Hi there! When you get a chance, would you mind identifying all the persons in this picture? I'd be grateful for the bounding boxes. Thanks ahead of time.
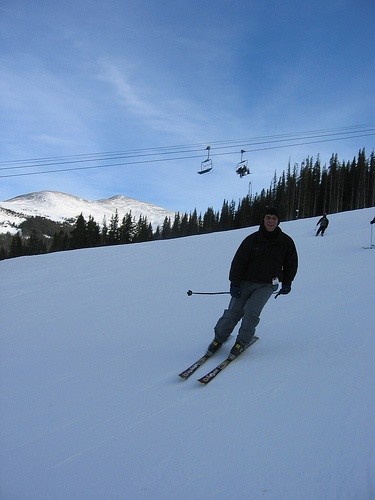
[237,165,252,176]
[206,206,298,358]
[316,214,329,236]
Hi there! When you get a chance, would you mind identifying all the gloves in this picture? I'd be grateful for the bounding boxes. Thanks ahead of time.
[279,281,291,295]
[230,284,241,298]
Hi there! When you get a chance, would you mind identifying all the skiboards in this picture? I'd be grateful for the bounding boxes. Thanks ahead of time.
[360,240,375,250]
[174,332,259,387]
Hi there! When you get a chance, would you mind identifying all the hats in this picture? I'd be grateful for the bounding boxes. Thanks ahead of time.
[262,206,280,225]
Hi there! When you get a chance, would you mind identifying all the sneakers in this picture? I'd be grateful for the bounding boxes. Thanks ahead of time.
[230,341,245,357]
[208,338,222,353]
